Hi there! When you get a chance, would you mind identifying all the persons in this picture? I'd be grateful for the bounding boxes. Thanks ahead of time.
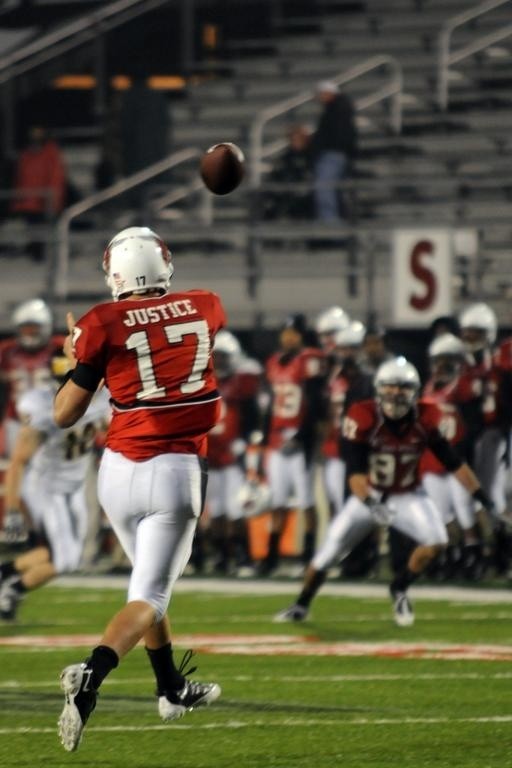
[294,74,357,225]
[10,117,64,262]
[1,223,511,752]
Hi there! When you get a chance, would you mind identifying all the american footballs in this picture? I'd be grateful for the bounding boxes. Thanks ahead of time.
[200,143,245,195]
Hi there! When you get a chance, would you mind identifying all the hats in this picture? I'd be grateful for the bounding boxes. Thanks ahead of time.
[279,313,306,334]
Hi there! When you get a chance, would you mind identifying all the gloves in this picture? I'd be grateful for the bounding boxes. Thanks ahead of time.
[369,491,397,525]
[0,513,29,545]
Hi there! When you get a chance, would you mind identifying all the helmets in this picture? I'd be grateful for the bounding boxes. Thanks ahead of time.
[212,330,239,379]
[238,479,273,518]
[11,298,52,353]
[374,356,423,416]
[428,302,498,380]
[102,225,175,302]
[314,305,386,358]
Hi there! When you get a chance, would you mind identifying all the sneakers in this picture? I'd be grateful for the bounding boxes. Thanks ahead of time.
[0,560,23,620]
[389,583,414,627]
[57,661,99,751]
[158,681,221,724]
[183,554,376,580]
[272,603,309,624]
[429,543,511,584]
